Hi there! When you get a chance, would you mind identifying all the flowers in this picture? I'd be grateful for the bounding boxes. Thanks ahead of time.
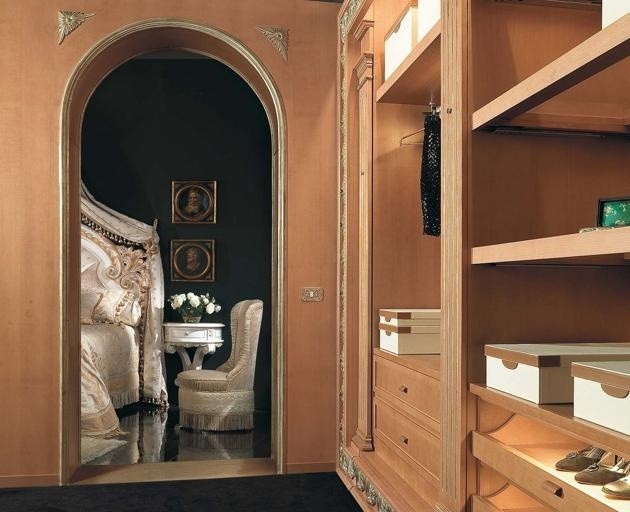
[167,292,222,317]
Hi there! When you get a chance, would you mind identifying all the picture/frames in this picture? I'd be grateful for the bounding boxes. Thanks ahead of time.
[171,179,217,225]
[170,240,215,282]
[598,197,630,225]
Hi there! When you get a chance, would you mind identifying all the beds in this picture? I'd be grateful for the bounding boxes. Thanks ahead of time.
[78,177,169,437]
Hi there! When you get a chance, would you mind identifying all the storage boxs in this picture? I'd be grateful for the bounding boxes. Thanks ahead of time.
[380,308,440,327]
[485,342,630,404]
[379,322,440,355]
[570,361,630,438]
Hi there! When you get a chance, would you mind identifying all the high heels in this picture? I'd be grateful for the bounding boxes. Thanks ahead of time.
[554,446,608,471]
[575,450,630,484]
[602,473,630,499]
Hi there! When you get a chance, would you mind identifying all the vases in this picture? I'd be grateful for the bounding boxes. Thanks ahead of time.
[182,318,201,322]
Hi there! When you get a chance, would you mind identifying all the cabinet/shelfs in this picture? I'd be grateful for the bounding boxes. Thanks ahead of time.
[452,0,630,512]
[336,0,450,512]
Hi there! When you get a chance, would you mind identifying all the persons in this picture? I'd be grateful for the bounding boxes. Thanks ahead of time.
[184,248,203,275]
[183,190,204,216]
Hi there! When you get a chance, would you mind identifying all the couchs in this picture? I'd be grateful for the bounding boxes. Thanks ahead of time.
[174,298,264,431]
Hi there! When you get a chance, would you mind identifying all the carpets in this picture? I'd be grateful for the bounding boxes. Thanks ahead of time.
[80,437,129,463]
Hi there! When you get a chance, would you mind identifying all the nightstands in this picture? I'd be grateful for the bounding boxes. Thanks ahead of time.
[163,319,225,386]
